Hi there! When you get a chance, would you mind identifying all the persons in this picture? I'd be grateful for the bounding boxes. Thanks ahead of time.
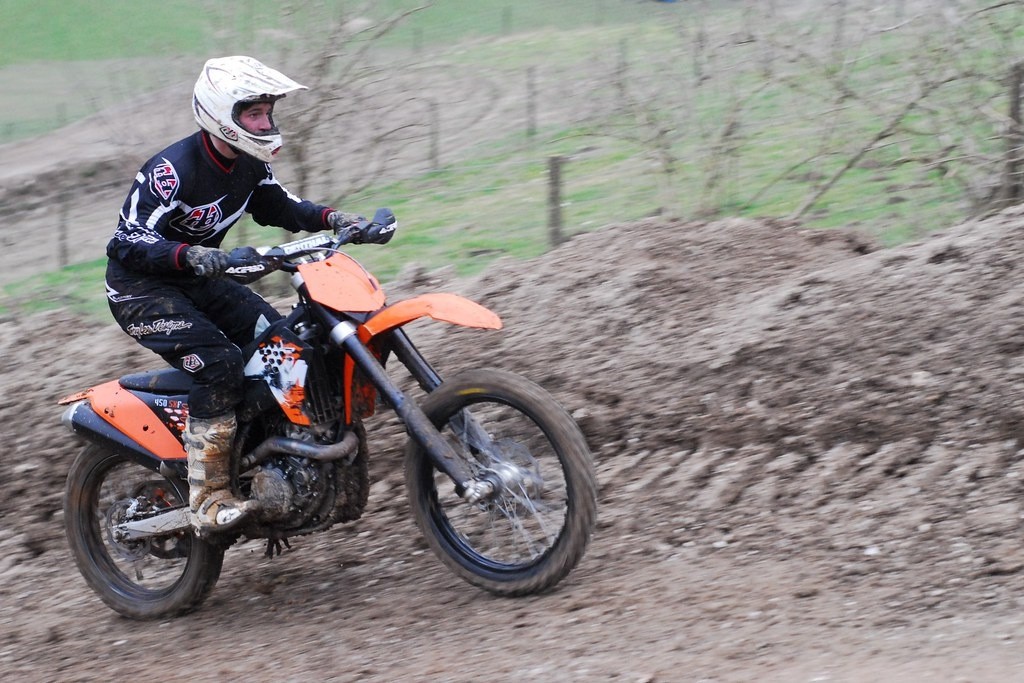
[105,56,368,530]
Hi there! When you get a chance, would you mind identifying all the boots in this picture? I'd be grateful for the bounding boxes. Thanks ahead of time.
[185,408,261,533]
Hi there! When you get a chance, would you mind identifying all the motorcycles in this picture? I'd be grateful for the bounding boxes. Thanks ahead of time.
[47,220,601,623]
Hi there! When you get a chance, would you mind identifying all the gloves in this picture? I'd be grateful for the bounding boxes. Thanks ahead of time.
[186,245,230,281]
[326,211,370,246]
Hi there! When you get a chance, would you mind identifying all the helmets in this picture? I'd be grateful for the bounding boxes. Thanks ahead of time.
[191,55,309,164]
